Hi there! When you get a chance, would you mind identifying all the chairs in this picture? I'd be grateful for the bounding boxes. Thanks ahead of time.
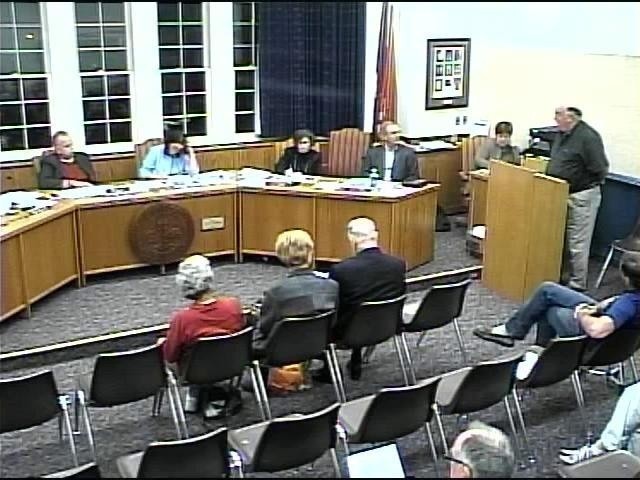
[593,212,640,288]
[459,135,496,202]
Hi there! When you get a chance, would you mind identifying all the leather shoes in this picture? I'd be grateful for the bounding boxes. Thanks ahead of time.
[346,359,362,380]
[557,444,609,466]
[472,323,515,347]
[310,367,344,384]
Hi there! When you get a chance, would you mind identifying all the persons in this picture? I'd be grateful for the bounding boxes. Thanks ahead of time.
[471,249,640,348]
[361,120,420,181]
[164,254,244,420]
[274,128,320,177]
[37,131,96,190]
[239,227,341,393]
[557,380,640,466]
[474,121,522,168]
[544,104,609,293]
[449,422,514,479]
[137,128,199,180]
[311,216,406,383]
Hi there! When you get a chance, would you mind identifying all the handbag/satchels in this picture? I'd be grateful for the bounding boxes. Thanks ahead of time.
[200,385,241,420]
[267,363,306,396]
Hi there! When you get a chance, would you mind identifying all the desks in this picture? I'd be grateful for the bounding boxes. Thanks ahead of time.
[466,167,490,256]
[417,149,469,215]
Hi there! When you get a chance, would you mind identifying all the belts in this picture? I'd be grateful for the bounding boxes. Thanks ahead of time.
[568,180,600,195]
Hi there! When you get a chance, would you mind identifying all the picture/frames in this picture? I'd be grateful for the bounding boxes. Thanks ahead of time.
[425,37,472,110]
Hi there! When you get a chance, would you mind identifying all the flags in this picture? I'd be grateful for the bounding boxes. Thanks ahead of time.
[372,2,398,140]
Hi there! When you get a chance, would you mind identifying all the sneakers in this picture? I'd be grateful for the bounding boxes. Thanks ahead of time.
[184,389,198,412]
